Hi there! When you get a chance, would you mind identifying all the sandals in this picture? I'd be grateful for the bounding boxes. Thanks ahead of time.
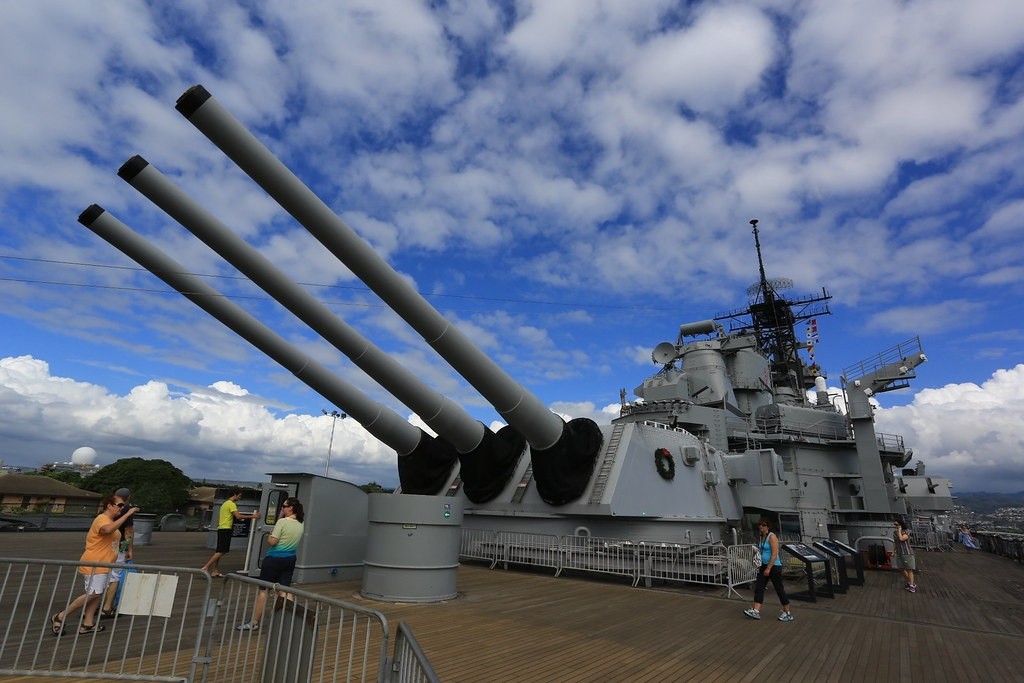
[101,609,121,618]
[236,621,259,630]
[51,611,66,636]
[79,624,105,634]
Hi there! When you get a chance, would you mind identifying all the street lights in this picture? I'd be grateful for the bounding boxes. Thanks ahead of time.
[322,409,348,479]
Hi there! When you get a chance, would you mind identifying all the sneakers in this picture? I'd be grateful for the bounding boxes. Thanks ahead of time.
[778,612,794,621]
[743,608,760,619]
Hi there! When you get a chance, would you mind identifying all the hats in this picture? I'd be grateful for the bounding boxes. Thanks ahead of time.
[115,488,130,499]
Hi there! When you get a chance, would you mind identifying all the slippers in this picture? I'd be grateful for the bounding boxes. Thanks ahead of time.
[211,573,225,578]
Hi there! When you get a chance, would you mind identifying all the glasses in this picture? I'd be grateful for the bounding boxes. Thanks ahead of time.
[894,523,900,526]
[283,505,294,509]
[110,503,124,507]
[759,523,767,527]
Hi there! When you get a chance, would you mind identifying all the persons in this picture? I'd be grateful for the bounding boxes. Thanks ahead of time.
[50,495,140,636]
[743,517,794,621]
[237,497,305,630]
[891,519,917,592]
[80,488,134,619]
[201,488,262,578]
[960,525,977,551]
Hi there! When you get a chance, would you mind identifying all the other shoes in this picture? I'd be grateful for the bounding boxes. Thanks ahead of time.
[905,582,916,593]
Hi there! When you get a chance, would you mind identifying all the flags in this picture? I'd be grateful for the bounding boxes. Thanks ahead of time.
[806,319,819,376]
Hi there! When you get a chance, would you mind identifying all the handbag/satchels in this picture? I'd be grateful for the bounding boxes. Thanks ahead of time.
[113,560,138,609]
